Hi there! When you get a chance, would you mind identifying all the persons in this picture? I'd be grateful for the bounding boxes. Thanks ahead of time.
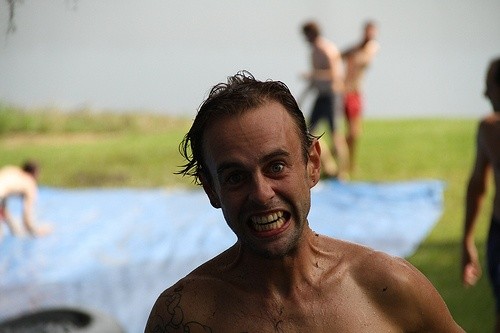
[462,57,500,333]
[339,18,379,182]
[144,70,468,333]
[1,158,53,238]
[298,21,350,183]
